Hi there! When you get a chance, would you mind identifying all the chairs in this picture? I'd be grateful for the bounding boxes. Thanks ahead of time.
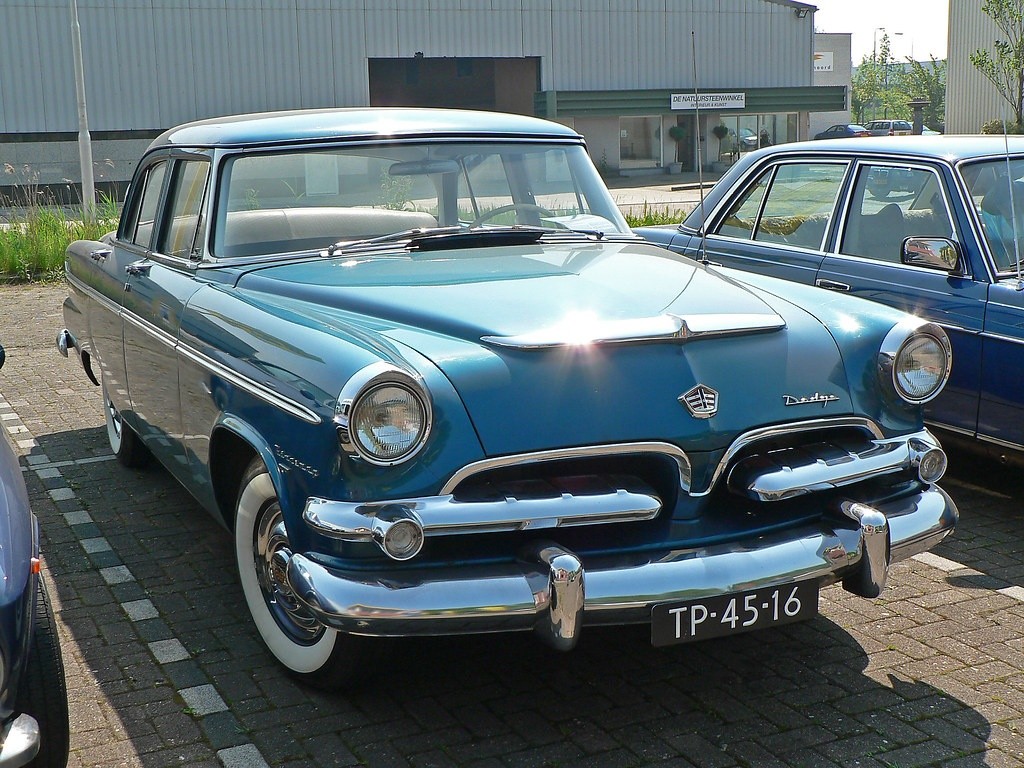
[864,203,908,262]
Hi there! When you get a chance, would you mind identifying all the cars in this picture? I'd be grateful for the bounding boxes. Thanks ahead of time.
[813,120,942,140]
[54,104,962,697]
[727,128,763,152]
[627,131,1024,501]
[1,341,72,768]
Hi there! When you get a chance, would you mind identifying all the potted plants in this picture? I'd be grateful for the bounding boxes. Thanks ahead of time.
[667,124,687,174]
[713,125,729,173]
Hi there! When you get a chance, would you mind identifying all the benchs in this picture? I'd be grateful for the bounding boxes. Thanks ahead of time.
[136,207,438,258]
[761,193,950,263]
